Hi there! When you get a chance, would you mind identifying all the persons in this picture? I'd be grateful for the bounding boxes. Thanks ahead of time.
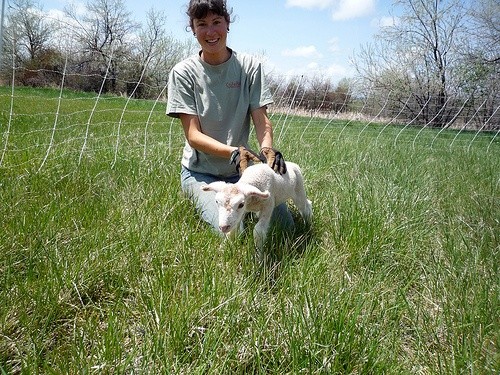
[166,0,294,246]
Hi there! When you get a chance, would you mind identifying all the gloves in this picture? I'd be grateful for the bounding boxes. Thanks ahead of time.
[228,145,261,179]
[258,147,287,175]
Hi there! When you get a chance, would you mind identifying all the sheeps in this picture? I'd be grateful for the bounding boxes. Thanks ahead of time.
[201,160,312,251]
